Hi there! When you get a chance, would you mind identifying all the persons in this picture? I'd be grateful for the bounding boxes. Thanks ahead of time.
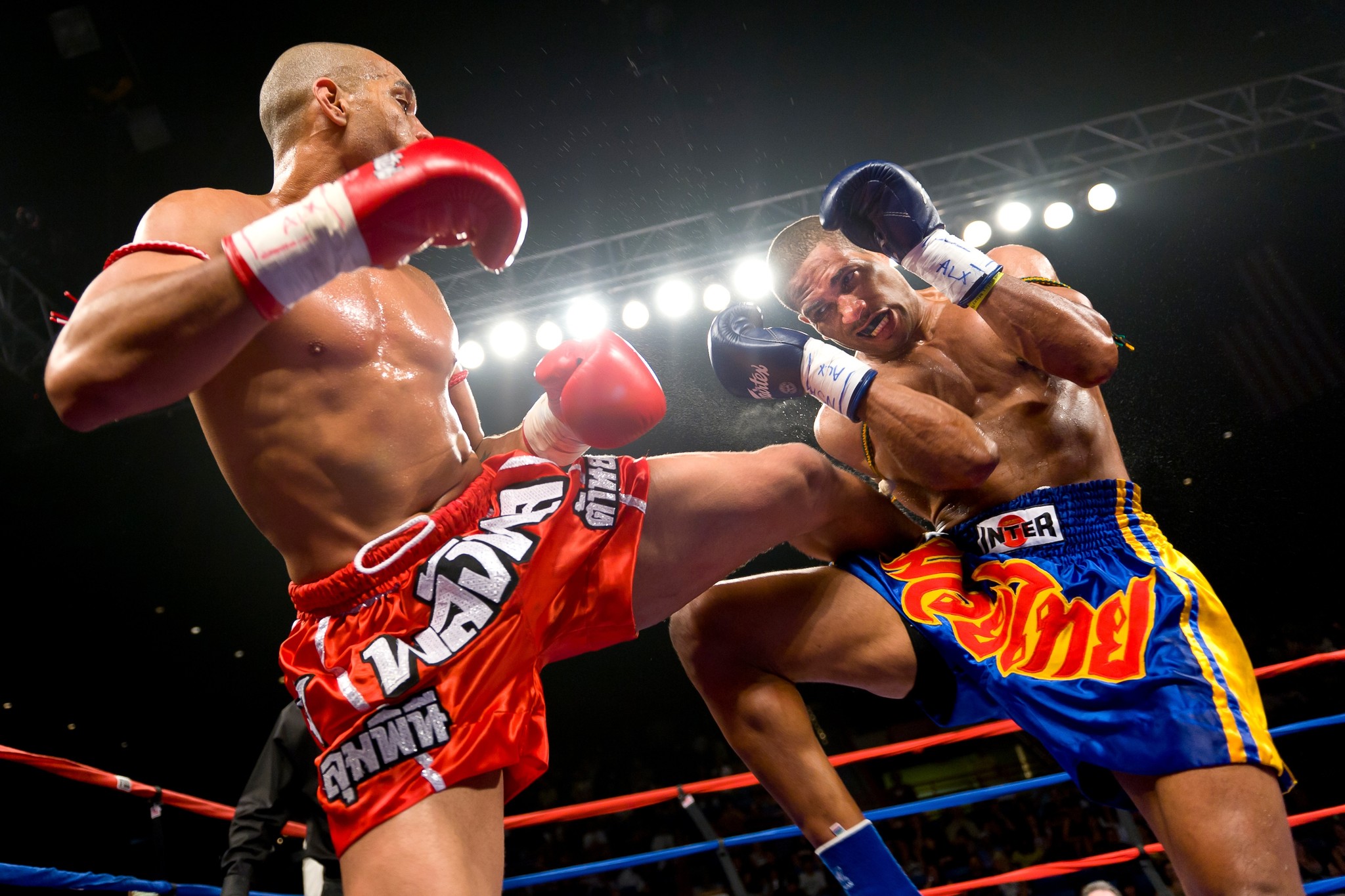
[505,608,1345,896]
[1082,880,1121,896]
[668,158,1307,896]
[43,41,929,896]
[221,699,343,896]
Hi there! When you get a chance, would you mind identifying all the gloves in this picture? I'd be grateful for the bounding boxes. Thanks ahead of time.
[519,329,668,468]
[709,304,879,424]
[819,160,1003,306]
[223,137,526,319]
